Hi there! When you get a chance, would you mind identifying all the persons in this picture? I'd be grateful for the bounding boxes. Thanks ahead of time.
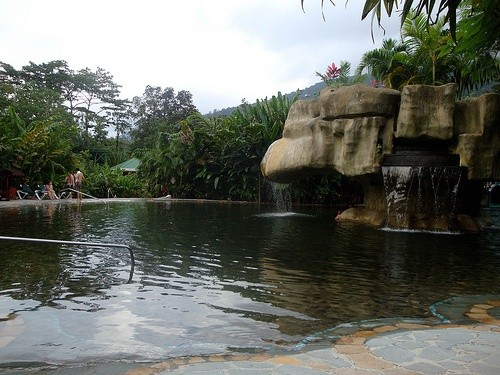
[45,181,55,200]
[64,171,74,199]
[75,168,84,200]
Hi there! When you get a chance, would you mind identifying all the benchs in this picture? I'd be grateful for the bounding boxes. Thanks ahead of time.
[17,184,66,200]
